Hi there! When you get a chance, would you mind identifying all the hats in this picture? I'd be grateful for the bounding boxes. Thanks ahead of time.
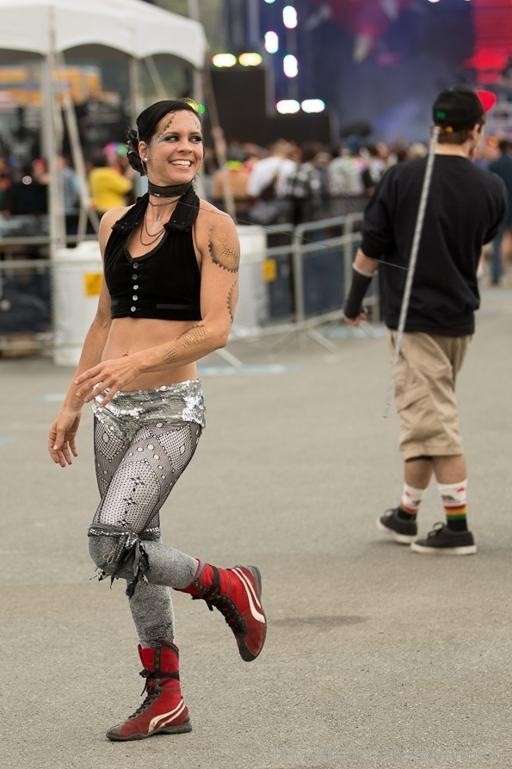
[432,86,497,132]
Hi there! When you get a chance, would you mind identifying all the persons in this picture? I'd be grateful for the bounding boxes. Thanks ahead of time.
[341,86,512,559]
[0,135,510,289]
[46,101,269,741]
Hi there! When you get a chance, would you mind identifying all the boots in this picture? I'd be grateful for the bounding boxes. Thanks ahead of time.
[107,637,192,740]
[172,559,267,661]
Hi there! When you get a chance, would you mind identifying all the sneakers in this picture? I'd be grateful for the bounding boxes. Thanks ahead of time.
[411,522,477,555]
[376,508,417,544]
